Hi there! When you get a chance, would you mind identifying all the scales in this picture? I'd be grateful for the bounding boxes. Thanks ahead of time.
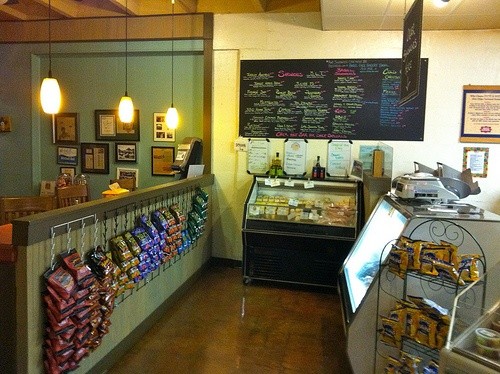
[394,171,461,204]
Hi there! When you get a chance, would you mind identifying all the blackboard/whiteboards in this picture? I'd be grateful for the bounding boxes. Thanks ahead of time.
[238,58,429,142]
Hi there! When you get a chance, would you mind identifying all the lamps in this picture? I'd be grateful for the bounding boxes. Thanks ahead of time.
[164,1,181,131]
[38,1,63,115]
[117,1,135,123]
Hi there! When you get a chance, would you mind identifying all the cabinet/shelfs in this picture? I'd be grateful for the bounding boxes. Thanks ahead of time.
[371,218,487,373]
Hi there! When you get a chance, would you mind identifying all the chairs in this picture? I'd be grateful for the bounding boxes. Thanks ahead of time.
[110,177,135,193]
[55,183,91,209]
[1,196,59,224]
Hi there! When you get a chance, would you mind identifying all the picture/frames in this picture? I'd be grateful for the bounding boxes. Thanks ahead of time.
[60,167,76,177]
[94,109,141,142]
[151,146,175,176]
[115,142,137,162]
[40,180,56,196]
[51,113,79,145]
[56,147,79,166]
[116,168,139,188]
[152,111,176,143]
[80,142,110,174]
[0,114,12,132]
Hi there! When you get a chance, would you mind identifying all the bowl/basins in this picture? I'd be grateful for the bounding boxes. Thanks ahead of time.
[474,341,500,360]
[475,327,500,347]
